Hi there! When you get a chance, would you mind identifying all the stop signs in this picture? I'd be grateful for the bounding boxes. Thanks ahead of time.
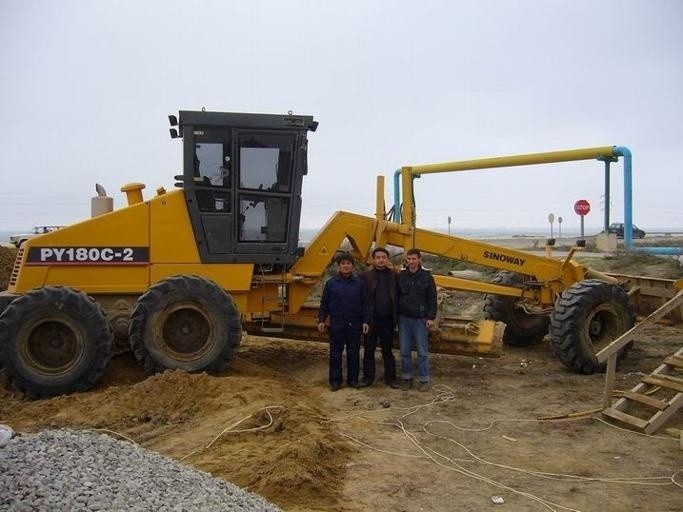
[574,199,590,216]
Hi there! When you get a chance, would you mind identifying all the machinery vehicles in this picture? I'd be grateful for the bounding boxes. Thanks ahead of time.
[0,108,682,401]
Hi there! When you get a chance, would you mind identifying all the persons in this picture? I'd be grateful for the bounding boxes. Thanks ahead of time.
[397,248,437,392]
[317,254,369,391]
[357,248,399,389]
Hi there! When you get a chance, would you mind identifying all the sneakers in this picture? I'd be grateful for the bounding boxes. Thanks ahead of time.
[359,379,370,386]
[331,383,339,391]
[386,380,399,388]
[420,382,429,391]
[402,380,412,391]
[350,380,360,388]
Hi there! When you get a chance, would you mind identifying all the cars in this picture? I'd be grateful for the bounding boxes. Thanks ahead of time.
[8,224,67,248]
[607,224,645,239]
